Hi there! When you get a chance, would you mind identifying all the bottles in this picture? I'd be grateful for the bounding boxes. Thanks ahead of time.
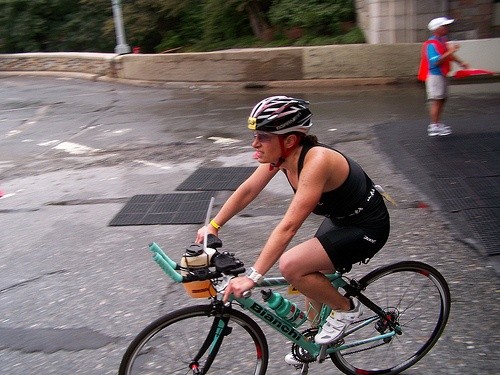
[180,242,216,299]
[260,288,307,327]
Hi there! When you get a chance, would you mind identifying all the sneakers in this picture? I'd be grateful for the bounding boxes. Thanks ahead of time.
[314,296,364,344]
[284,348,308,365]
[428,123,452,136]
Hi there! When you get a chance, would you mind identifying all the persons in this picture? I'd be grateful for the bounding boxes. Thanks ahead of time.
[417,17,468,135]
[195,96,390,365]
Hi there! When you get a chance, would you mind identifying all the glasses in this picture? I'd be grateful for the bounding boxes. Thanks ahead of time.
[252,132,274,142]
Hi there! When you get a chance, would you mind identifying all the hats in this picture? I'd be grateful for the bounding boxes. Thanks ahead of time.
[427,17,454,31]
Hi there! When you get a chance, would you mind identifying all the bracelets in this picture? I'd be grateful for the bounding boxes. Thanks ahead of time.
[210,220,221,229]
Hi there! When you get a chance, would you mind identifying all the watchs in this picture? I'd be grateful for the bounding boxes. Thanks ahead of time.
[245,267,264,285]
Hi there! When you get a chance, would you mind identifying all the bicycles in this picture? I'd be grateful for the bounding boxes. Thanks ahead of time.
[118,233,451,375]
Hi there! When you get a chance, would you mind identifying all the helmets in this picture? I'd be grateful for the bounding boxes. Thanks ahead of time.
[248,95,312,134]
